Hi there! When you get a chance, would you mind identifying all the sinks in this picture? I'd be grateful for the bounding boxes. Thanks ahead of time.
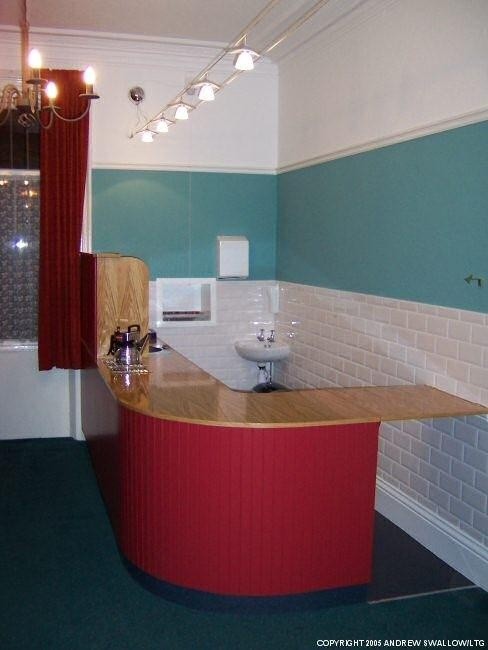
[235,339,289,361]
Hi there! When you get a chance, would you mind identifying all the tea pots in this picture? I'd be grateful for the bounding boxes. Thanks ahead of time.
[113,342,141,366]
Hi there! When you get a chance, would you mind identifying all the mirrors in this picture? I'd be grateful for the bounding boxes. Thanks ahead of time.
[154,277,217,327]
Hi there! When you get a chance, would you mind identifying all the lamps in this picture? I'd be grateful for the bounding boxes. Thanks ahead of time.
[135,34,261,142]
[1,0,101,132]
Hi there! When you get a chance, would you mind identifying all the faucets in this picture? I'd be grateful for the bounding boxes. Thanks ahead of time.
[267,330,276,342]
[256,329,264,341]
